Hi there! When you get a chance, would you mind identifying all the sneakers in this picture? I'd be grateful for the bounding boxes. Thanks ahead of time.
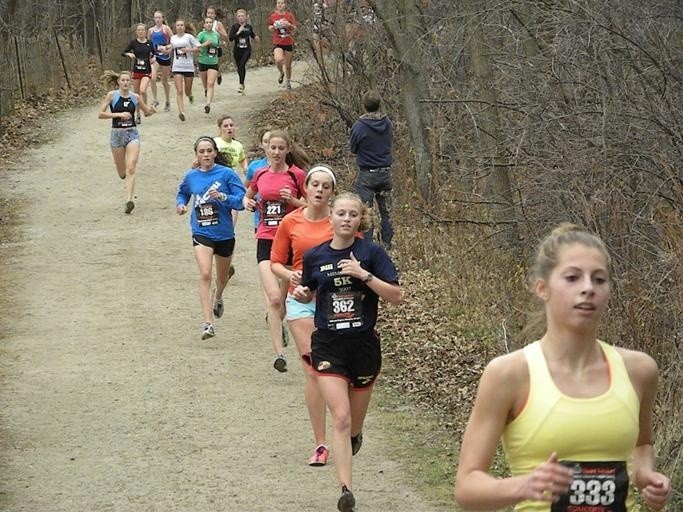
[309,444,329,467]
[286,82,291,90]
[216,74,223,85]
[350,425,364,456]
[278,72,284,84]
[237,84,246,93]
[204,89,208,97]
[135,111,143,124]
[189,96,195,104]
[228,264,236,280]
[211,287,225,319]
[150,100,160,112]
[178,111,186,121]
[265,312,290,348]
[205,104,211,114]
[337,483,356,512]
[201,321,215,341]
[125,199,135,215]
[272,353,288,374]
[163,101,171,112]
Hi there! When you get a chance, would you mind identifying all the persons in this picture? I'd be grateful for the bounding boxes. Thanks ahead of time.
[455,220,671,512]
[270,163,367,466]
[229,8,260,93]
[304,0,331,71]
[120,5,230,120]
[176,129,247,341]
[292,193,403,512]
[192,116,248,282]
[97,70,156,214]
[267,0,297,89]
